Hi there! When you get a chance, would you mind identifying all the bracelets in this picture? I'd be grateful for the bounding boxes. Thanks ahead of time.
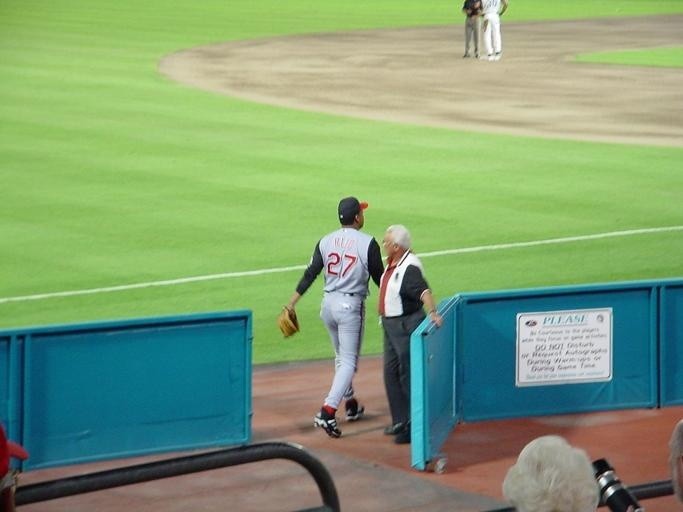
[428,309,437,315]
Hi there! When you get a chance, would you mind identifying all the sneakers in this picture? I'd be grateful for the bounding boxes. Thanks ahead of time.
[462,53,501,62]
[313,406,342,438]
[346,404,364,421]
[385,423,402,435]
[395,428,411,443]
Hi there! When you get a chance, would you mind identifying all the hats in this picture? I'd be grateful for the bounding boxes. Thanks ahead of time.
[338,197,369,220]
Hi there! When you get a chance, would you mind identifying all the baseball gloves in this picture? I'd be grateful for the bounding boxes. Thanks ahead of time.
[278,306,298,337]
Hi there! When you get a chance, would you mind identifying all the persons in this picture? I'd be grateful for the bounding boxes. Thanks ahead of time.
[463,0,483,59]
[501,435,601,512]
[626,421,683,511]
[480,0,509,61]
[378,224,443,443]
[290,197,385,439]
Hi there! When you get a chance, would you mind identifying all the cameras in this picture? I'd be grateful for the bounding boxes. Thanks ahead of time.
[592,456,646,512]
